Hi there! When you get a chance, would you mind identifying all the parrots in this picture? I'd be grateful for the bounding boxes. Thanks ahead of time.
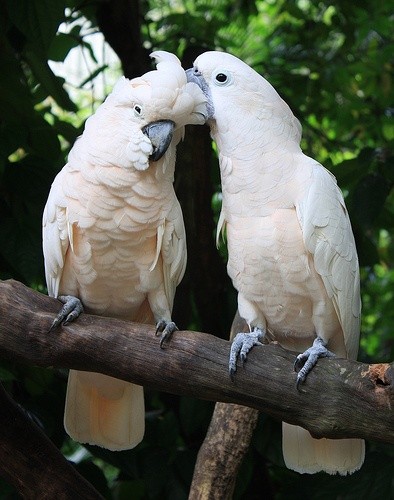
[42,51,208,452]
[185,49,365,475]
